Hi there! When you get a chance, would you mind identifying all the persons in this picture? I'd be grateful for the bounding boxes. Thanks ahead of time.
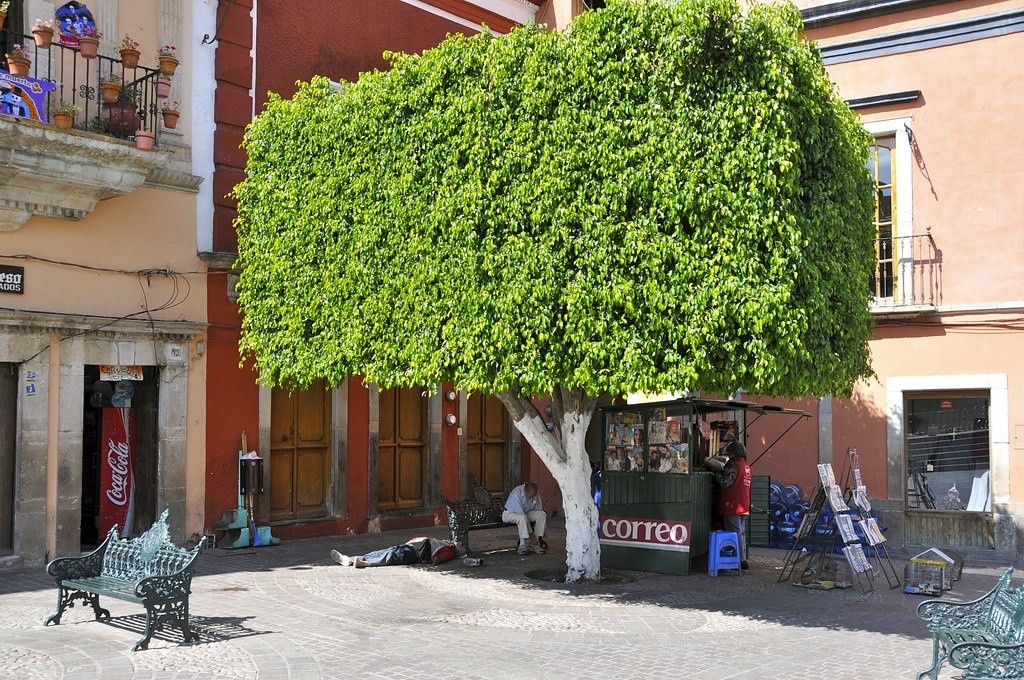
[650,450,689,473]
[606,449,644,471]
[634,429,644,445]
[666,421,681,444]
[648,424,659,444]
[941,488,964,510]
[716,442,752,570]
[331,537,456,567]
[609,424,630,445]
[502,482,548,554]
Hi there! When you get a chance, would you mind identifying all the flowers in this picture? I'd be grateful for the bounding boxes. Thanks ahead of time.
[95,70,144,107]
[81,25,103,41]
[156,46,177,59]
[118,34,140,50]
[35,18,55,27]
[100,72,121,85]
[161,98,182,111]
[2,44,32,66]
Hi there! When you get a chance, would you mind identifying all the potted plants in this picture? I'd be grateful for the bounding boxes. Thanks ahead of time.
[0,1,10,30]
[153,72,174,99]
[50,98,83,128]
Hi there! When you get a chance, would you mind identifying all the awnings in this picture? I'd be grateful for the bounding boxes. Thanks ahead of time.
[694,398,813,470]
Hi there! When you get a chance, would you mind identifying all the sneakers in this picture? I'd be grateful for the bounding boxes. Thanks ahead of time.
[528,543,546,555]
[519,544,527,555]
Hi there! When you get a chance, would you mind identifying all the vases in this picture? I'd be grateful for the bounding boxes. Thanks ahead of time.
[101,82,121,103]
[5,55,32,79]
[119,49,141,69]
[32,24,54,49]
[162,110,180,129]
[100,102,141,139]
[134,131,155,152]
[77,37,100,59]
[158,57,178,75]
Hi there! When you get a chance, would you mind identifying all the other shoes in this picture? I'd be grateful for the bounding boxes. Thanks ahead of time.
[740,561,748,570]
[354,557,362,569]
[330,550,352,566]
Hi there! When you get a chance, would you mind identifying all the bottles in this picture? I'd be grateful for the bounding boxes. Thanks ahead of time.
[768,482,884,556]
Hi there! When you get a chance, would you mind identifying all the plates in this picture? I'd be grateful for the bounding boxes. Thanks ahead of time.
[84,379,134,407]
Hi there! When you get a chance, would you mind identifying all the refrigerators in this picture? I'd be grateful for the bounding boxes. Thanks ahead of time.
[80,405,138,546]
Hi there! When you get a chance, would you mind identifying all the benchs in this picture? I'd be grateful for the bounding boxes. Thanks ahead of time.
[44,508,207,650]
[440,473,548,566]
[915,565,1024,680]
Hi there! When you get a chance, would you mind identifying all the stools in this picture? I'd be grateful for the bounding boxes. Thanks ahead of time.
[707,530,743,577]
